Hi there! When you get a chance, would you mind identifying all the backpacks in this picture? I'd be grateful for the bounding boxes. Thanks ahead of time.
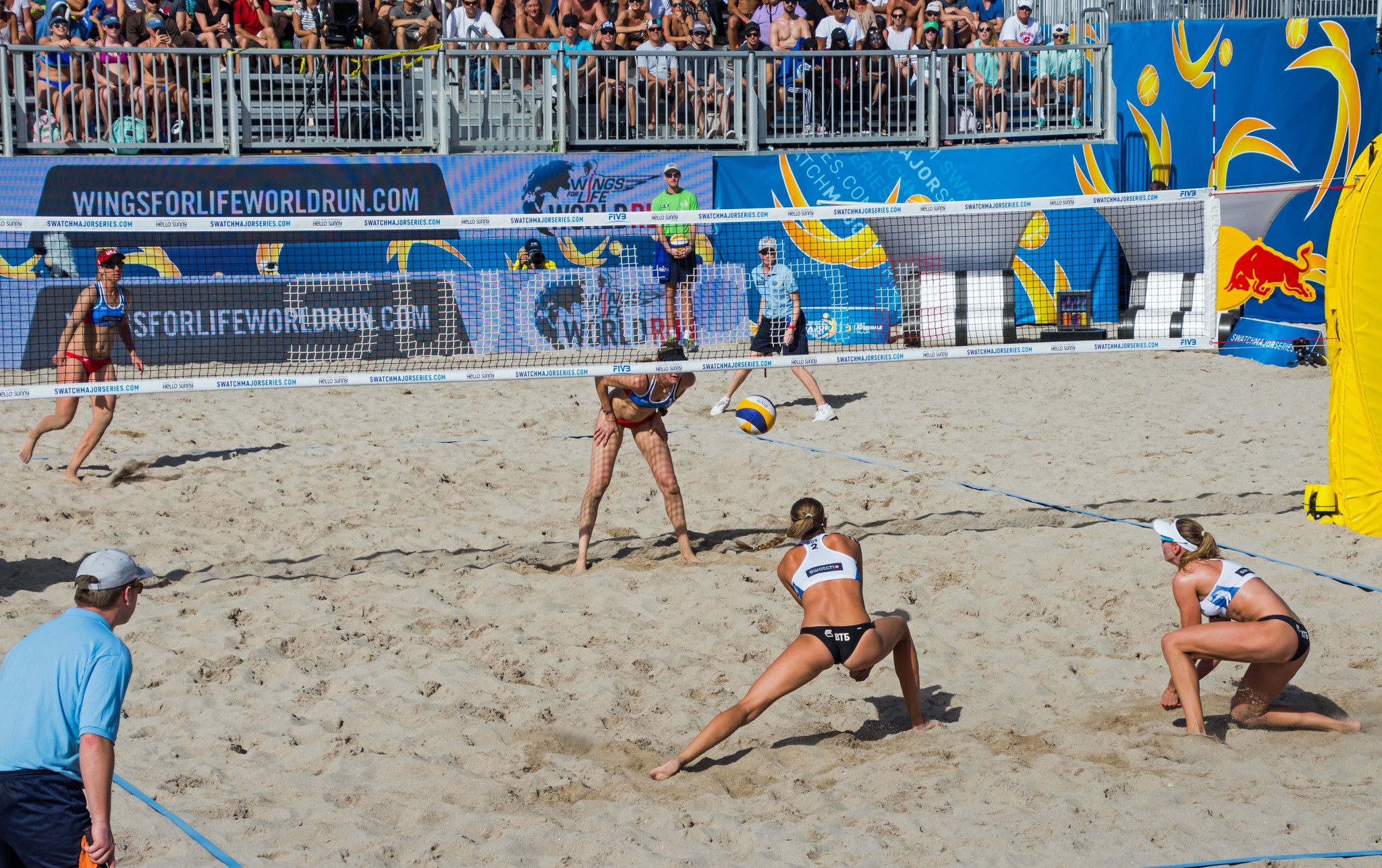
[953,105,979,133]
[108,117,145,155]
[26,110,68,155]
[467,58,500,90]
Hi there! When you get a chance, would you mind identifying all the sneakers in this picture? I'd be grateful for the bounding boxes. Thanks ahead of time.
[668,335,699,352]
[812,405,836,422]
[710,397,730,416]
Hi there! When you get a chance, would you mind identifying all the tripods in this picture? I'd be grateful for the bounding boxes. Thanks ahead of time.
[283,45,413,144]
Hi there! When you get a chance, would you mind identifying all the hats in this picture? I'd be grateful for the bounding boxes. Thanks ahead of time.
[102,16,120,25]
[758,236,775,251]
[524,238,542,252]
[562,14,579,25]
[1019,0,1033,9]
[1153,518,1197,552]
[600,21,616,30]
[833,0,849,8]
[745,21,760,32]
[146,19,165,29]
[924,21,939,31]
[692,21,708,34]
[97,250,126,271]
[1052,24,1068,34]
[75,548,154,590]
[648,19,662,28]
[832,28,848,39]
[664,162,680,173]
[50,15,68,26]
[925,2,941,12]
[658,348,688,361]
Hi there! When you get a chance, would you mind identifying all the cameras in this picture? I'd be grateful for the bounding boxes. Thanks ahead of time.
[523,249,547,266]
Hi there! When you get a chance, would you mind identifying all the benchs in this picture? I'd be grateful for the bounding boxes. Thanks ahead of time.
[0,0,1090,134]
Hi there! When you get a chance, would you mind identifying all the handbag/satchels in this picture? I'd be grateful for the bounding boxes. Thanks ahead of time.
[602,121,633,140]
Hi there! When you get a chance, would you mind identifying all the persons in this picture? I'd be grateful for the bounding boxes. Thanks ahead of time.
[1153,518,1366,737]
[647,498,946,784]
[0,547,154,868]
[1,0,1083,576]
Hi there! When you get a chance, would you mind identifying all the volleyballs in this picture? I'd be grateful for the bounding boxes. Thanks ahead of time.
[669,234,689,252]
[736,395,777,436]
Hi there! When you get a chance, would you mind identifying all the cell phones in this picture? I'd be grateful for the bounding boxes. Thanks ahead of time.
[159,27,166,38]
[412,30,422,38]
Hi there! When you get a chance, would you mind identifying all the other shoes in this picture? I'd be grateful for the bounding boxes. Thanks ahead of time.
[939,117,1081,146]
[404,68,437,79]
[275,63,290,82]
[599,121,736,139]
[767,99,893,135]
[502,79,510,90]
[220,66,235,74]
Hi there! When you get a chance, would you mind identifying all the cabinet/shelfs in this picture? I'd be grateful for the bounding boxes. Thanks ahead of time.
[1056,289,1094,331]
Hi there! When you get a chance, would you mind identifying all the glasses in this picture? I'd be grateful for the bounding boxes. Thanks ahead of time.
[565,23,578,28]
[666,173,680,178]
[746,32,759,38]
[760,250,775,255]
[629,0,641,3]
[870,36,882,41]
[92,6,102,11]
[102,260,124,269]
[54,7,67,12]
[869,29,881,34]
[1018,6,1031,12]
[464,0,477,6]
[149,0,160,3]
[122,582,144,594]
[53,22,68,28]
[892,14,904,18]
[1053,34,1066,38]
[107,24,120,29]
[785,0,796,5]
[672,3,684,8]
[835,5,847,10]
[924,29,936,34]
[603,30,615,34]
[927,12,940,16]
[649,27,663,32]
[979,26,991,32]
